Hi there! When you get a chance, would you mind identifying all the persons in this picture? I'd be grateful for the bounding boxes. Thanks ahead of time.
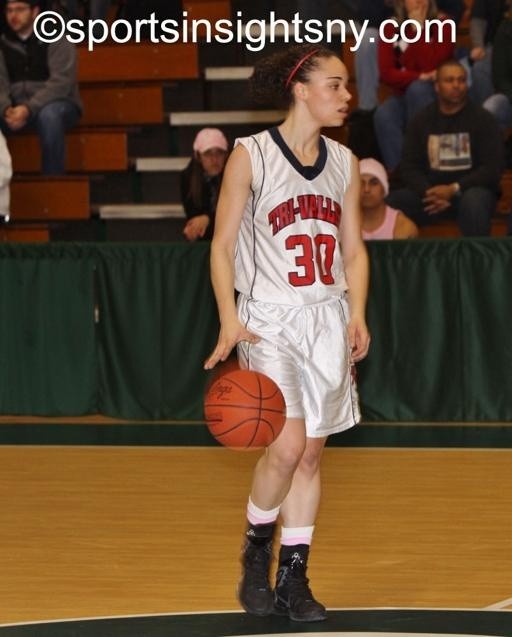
[0,0,87,176]
[349,156,422,239]
[382,57,507,236]
[199,39,375,626]
[48,0,189,42]
[348,1,511,188]
[177,126,232,242]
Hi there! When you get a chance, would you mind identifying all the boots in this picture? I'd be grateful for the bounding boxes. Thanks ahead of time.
[239,520,328,622]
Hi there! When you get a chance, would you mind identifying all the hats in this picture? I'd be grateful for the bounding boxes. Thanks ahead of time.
[358,157,390,199]
[192,127,228,155]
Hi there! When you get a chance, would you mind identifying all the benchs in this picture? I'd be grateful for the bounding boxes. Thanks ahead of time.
[0,2,512,242]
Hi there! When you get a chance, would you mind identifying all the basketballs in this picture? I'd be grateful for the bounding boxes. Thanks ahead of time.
[204,370,286,449]
